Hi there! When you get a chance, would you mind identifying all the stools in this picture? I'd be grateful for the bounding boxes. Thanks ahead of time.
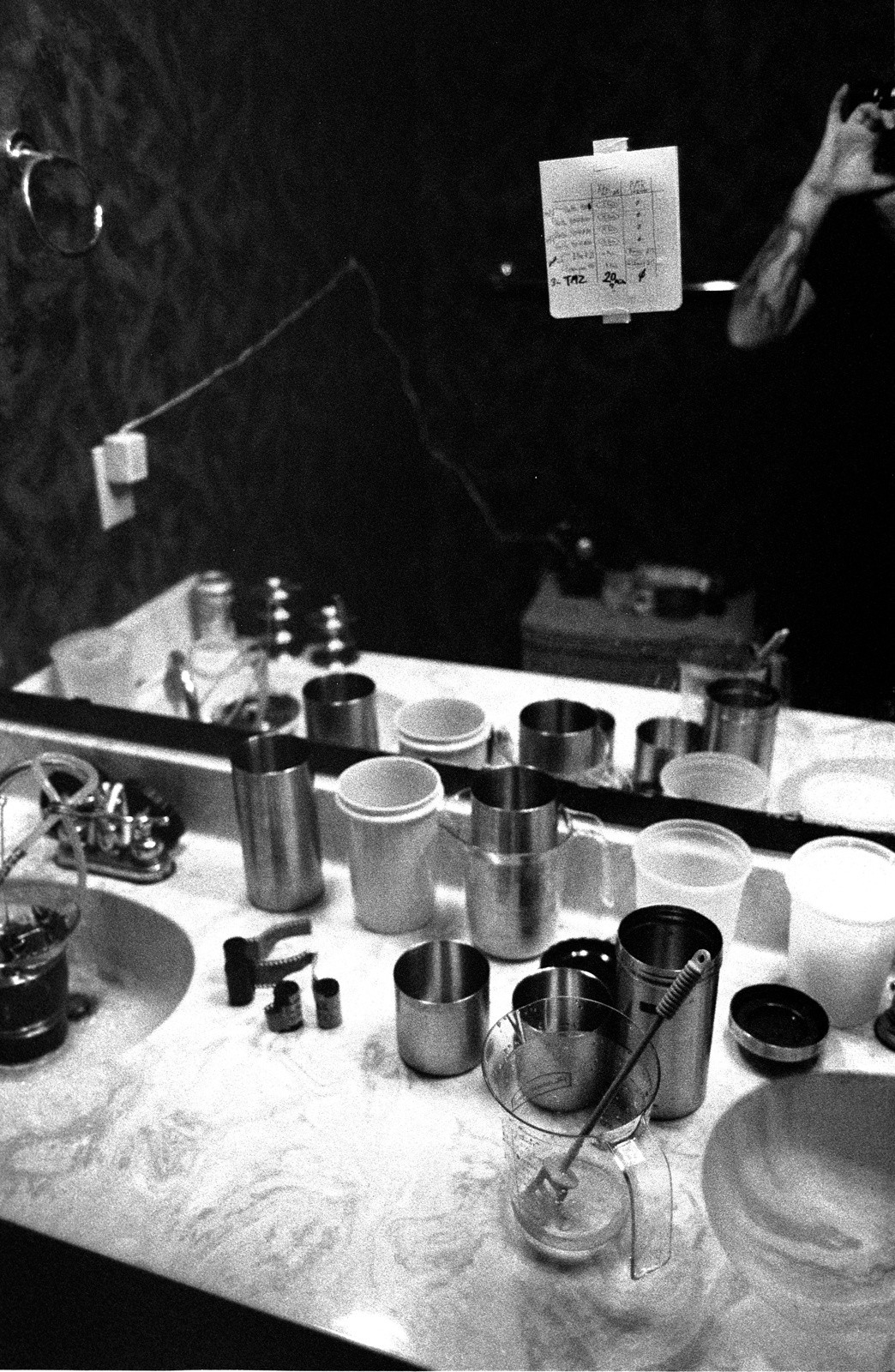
[519,559,767,693]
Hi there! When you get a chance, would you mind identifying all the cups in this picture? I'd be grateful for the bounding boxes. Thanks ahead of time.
[50,627,132,708]
[334,755,443,933]
[230,734,325,911]
[632,818,753,954]
[785,835,895,1030]
[519,698,616,772]
[613,904,724,1120]
[469,765,559,959]
[704,677,782,773]
[634,716,712,797]
[778,756,895,833]
[393,696,490,771]
[659,750,774,811]
[548,525,610,599]
[631,564,712,621]
[393,941,492,1077]
[511,967,612,1114]
[302,673,379,751]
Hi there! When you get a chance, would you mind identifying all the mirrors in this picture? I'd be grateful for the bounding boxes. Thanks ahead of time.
[0,0,895,861]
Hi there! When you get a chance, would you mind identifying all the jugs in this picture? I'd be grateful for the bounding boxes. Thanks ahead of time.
[438,787,614,959]
[678,636,782,728]
[481,996,673,1281]
[499,731,634,791]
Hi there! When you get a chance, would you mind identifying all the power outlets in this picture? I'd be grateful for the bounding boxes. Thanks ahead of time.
[88,442,139,534]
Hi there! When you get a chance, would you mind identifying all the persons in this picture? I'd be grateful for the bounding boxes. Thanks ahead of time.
[727,82,895,723]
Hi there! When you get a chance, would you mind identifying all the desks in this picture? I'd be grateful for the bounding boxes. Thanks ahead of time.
[0,793,895,1372]
[127,637,895,832]
